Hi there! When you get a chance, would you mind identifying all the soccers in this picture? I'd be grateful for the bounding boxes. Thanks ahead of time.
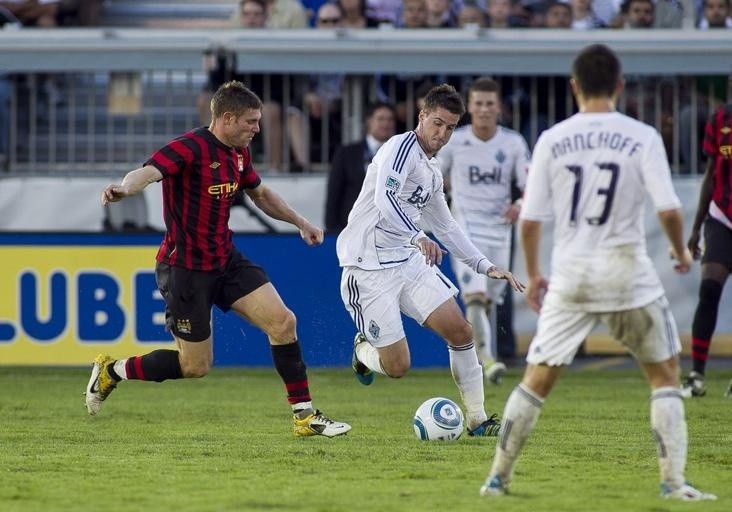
[414,397,464,440]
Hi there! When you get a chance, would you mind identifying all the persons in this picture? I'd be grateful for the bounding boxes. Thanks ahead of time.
[85,80,352,439]
[478,44,719,502]
[432,78,532,386]
[4,3,732,233]
[687,100,732,398]
[336,82,525,438]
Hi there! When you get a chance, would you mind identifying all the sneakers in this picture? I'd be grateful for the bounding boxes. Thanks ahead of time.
[483,360,507,388]
[478,471,513,498]
[675,369,707,400]
[658,480,719,504]
[466,413,503,438]
[352,329,377,387]
[292,409,353,438]
[82,352,118,415]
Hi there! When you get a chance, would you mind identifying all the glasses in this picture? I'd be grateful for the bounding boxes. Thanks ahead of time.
[318,18,343,25]
